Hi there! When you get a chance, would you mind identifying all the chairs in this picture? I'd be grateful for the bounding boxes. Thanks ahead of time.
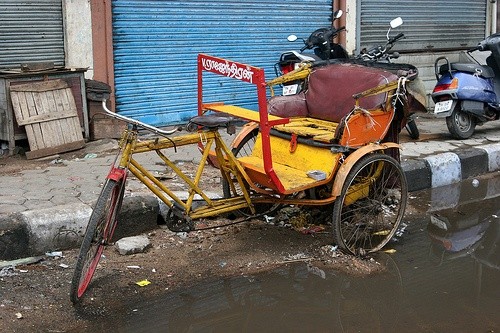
[267,63,398,144]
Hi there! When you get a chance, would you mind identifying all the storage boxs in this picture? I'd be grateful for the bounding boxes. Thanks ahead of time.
[86,79,128,139]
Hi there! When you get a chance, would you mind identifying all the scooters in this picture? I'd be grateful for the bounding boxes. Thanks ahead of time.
[431,30,500,138]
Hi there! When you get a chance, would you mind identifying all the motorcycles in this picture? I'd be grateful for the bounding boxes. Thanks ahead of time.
[351,15,420,140]
[274,7,349,96]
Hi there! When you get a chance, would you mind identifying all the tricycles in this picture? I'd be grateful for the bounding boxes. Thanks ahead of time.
[69,50,429,305]
